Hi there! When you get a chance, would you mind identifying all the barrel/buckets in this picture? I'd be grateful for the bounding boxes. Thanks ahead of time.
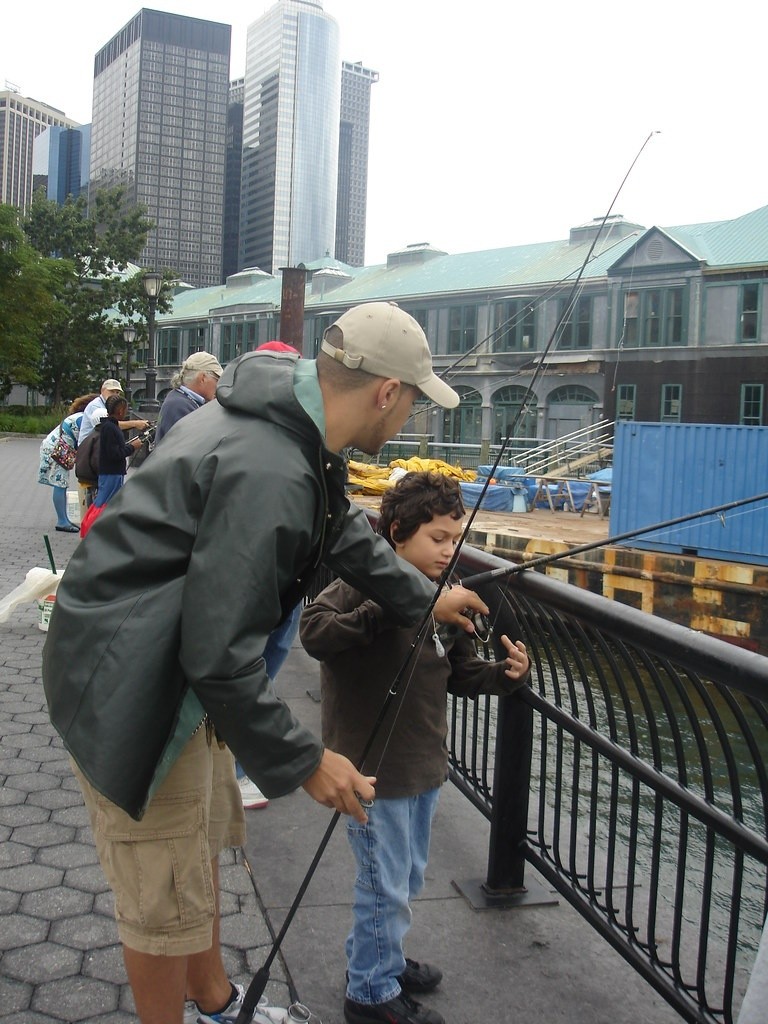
[38,570,65,631]
[66,490,81,523]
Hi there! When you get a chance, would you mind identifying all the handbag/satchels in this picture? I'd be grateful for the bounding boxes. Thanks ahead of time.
[51,437,78,471]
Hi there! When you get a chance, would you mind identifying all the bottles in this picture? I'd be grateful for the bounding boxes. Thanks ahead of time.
[281,1001,312,1024]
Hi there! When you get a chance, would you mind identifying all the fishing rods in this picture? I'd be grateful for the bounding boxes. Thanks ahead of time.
[449,493,768,645]
[123,211,644,450]
[222,129,666,1024]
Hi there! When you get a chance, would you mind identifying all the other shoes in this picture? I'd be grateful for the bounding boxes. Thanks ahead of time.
[70,521,82,531]
[237,774,269,809]
[55,523,79,533]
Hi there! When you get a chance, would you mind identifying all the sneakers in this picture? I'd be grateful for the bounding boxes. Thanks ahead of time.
[194,981,288,1024]
[344,990,445,1024]
[346,956,443,990]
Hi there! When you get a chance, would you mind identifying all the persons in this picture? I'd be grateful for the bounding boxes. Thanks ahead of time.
[297,472,532,1023]
[42,300,490,1024]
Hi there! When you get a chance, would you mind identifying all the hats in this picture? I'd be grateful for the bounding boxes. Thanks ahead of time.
[185,351,223,377]
[321,300,461,409]
[102,379,124,392]
[254,341,302,359]
